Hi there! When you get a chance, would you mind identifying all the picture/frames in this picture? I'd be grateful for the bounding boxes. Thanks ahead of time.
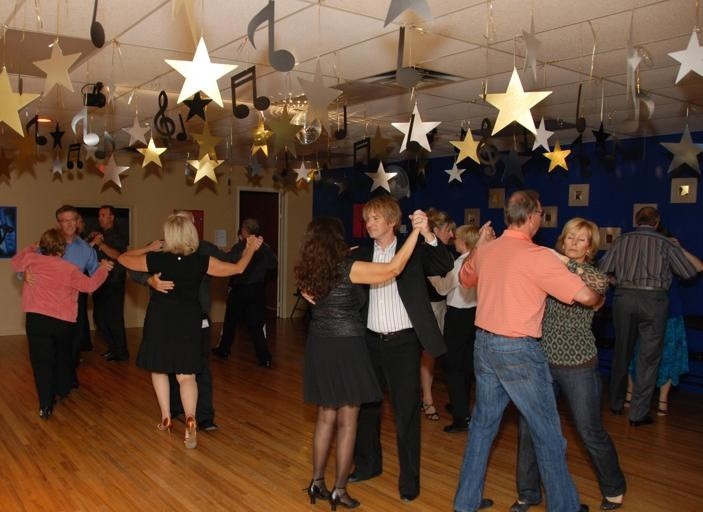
[463,177,700,251]
[72,205,132,248]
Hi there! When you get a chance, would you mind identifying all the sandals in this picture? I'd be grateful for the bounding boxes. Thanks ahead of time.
[612,392,669,425]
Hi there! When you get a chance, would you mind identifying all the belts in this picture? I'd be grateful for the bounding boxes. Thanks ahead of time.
[365,329,414,341]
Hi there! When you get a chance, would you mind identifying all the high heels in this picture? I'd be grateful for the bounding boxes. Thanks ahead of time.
[39,400,52,420]
[157,417,218,449]
[308,468,382,511]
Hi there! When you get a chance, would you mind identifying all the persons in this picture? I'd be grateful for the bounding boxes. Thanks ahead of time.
[294,190,700,512]
[12,205,130,419]
[117,210,277,448]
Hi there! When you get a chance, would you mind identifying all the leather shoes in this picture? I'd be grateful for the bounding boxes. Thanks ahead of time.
[454,496,623,512]
[258,360,271,367]
[211,347,228,360]
[100,349,129,361]
[421,403,471,433]
